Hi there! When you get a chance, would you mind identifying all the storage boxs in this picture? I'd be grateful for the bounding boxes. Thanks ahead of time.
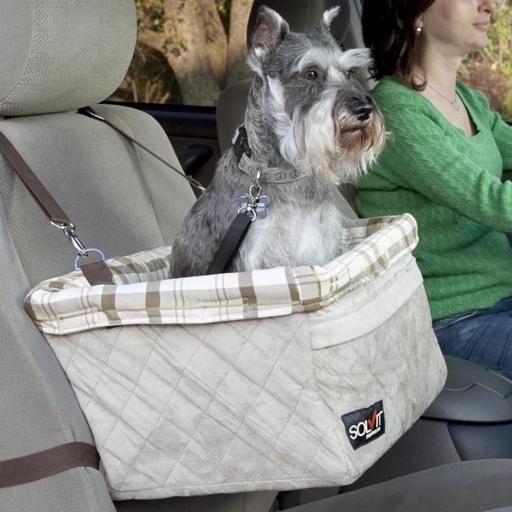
[27,214,446,501]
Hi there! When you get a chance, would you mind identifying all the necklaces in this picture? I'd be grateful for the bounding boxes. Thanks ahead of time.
[415,71,462,113]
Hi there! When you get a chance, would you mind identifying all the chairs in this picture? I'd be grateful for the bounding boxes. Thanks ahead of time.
[0,2,512,511]
[217,0,511,425]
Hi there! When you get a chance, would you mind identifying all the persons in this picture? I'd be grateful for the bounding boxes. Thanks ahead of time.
[347,0,512,384]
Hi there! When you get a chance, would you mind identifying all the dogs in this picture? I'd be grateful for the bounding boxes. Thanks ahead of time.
[168,6,395,279]
